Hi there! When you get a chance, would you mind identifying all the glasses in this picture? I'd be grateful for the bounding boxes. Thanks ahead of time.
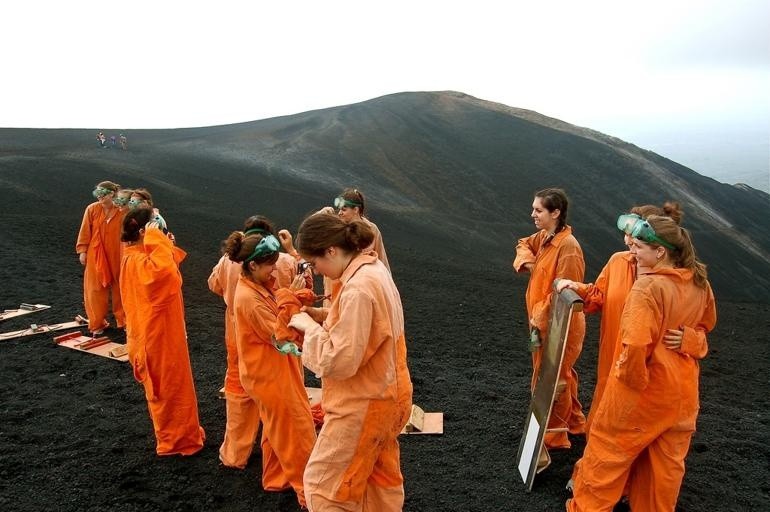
[150,214,167,231]
[255,233,280,255]
[617,214,642,236]
[127,199,143,210]
[270,333,301,357]
[92,188,111,198]
[333,196,361,209]
[113,196,129,206]
[632,219,673,251]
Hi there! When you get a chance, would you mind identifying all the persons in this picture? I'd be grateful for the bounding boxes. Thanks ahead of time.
[553,200,712,505]
[563,213,719,511]
[119,133,128,151]
[99,132,108,148]
[512,187,590,452]
[76,180,167,344]
[95,132,100,141]
[116,209,206,459]
[205,189,412,511]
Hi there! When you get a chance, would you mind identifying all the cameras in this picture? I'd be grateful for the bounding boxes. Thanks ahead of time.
[296,259,310,275]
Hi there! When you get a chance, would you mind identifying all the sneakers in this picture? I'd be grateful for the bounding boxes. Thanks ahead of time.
[93,329,105,338]
